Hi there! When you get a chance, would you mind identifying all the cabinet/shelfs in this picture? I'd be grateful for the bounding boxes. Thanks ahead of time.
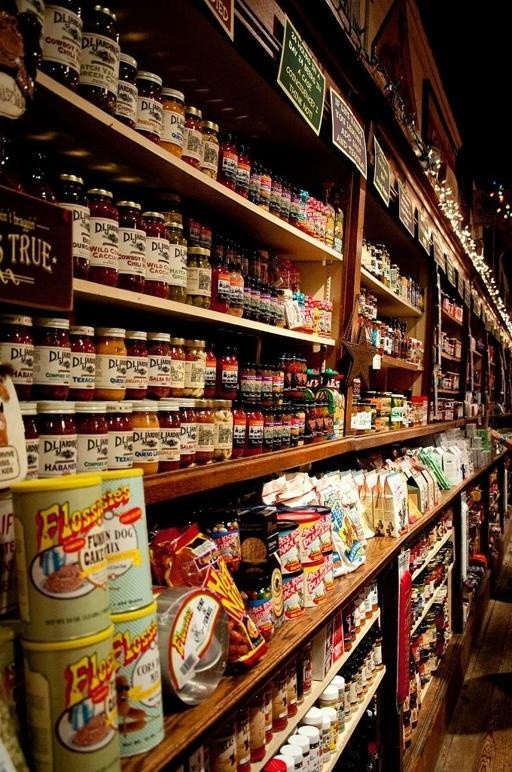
[0,0,512,772]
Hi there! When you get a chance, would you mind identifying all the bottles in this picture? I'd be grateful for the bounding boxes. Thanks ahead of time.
[27,151,55,202]
[0,136,22,190]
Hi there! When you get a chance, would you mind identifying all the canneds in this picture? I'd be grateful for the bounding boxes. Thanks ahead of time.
[354,389,409,435]
[187,246,212,310]
[144,212,170,300]
[175,399,198,466]
[161,87,186,157]
[97,326,126,401]
[185,340,205,398]
[204,343,217,397]
[124,330,150,398]
[244,276,285,329]
[80,8,120,114]
[212,398,234,460]
[19,402,38,482]
[36,400,78,479]
[211,576,381,772]
[137,70,163,143]
[164,221,187,303]
[196,399,216,464]
[0,313,36,400]
[148,332,171,398]
[42,0,80,92]
[8,476,105,644]
[21,0,45,56]
[87,189,118,288]
[218,345,237,398]
[228,261,245,320]
[210,253,229,316]
[168,337,185,397]
[14,625,124,771]
[157,402,182,468]
[108,604,164,756]
[116,53,139,128]
[361,287,414,360]
[83,469,154,618]
[233,404,306,449]
[133,400,160,475]
[240,352,326,406]
[403,511,451,736]
[35,316,72,397]
[107,404,133,470]
[199,119,219,178]
[75,401,107,472]
[361,237,425,310]
[182,105,204,170]
[69,326,95,400]
[220,132,336,250]
[187,218,213,248]
[116,201,146,295]
[55,174,91,281]
